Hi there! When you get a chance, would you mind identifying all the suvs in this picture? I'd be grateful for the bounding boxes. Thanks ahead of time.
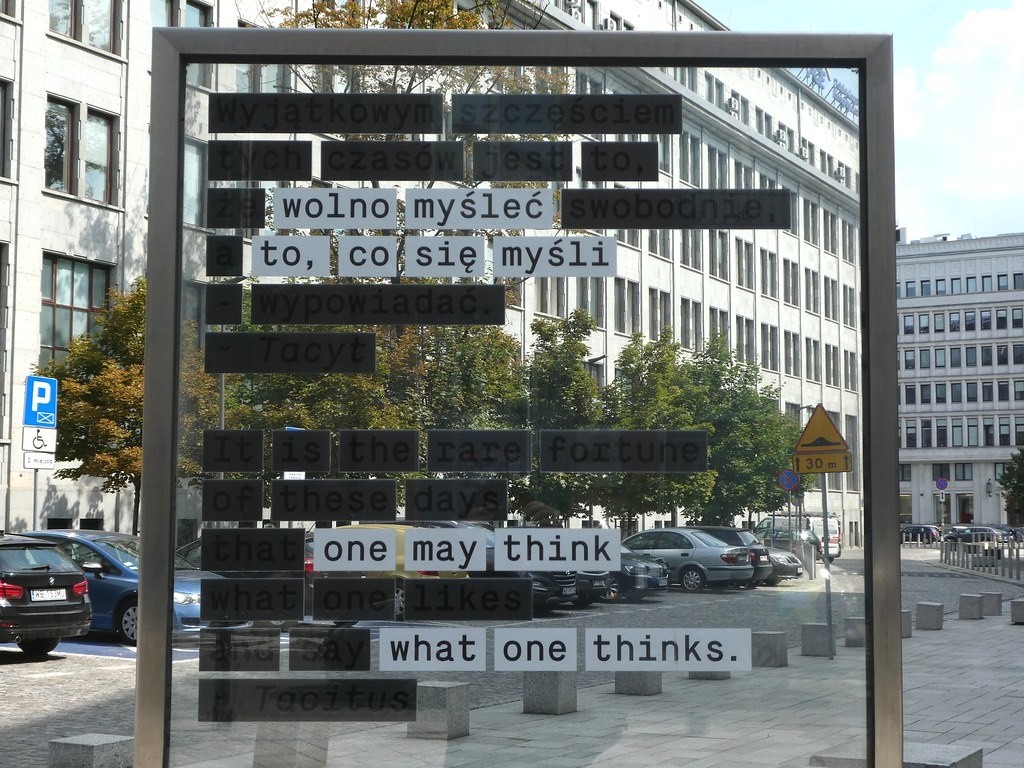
[754,529,823,561]
[0,531,93,656]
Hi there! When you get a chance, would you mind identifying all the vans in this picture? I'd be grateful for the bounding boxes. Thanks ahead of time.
[754,510,841,563]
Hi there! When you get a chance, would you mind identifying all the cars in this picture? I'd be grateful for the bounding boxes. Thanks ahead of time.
[943,523,1024,542]
[620,526,804,588]
[900,526,941,542]
[15,529,226,645]
[176,520,668,615]
